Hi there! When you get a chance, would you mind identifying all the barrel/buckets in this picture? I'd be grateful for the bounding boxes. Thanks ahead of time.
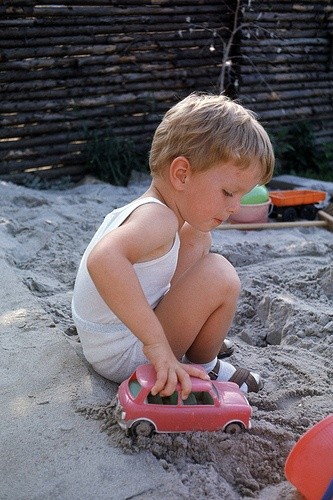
[283,415,333,500]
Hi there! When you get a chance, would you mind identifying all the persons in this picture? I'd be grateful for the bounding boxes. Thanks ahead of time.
[71,92,275,391]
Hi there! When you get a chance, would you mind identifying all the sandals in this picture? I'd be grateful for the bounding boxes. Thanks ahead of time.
[217,340,234,358]
[207,359,264,393]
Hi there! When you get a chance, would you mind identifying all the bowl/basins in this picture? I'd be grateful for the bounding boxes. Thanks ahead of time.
[228,197,272,224]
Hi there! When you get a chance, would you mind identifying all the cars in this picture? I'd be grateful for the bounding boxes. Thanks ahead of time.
[112,363,252,439]
